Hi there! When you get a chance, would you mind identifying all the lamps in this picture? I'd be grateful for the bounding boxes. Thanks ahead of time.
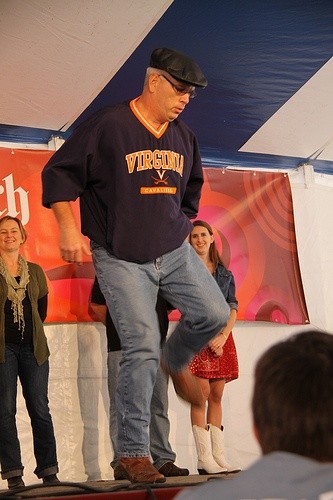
[48,133,65,151]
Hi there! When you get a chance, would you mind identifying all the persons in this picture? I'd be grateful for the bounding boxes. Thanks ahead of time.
[42,47,231,483]
[0,216,61,492]
[182,220,241,476]
[169,329,333,499]
[89,271,190,480]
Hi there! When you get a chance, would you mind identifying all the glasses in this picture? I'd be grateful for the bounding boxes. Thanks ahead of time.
[157,74,198,99]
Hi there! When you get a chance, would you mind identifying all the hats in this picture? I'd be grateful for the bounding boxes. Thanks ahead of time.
[149,47,207,89]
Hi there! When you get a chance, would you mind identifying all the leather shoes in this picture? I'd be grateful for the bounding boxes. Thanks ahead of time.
[113,457,166,484]
[160,359,204,406]
[158,461,189,477]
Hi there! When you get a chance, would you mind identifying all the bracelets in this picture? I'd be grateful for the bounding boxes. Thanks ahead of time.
[220,333,227,340]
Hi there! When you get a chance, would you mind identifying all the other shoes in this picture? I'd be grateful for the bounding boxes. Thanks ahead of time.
[7,476,25,489]
[42,474,60,485]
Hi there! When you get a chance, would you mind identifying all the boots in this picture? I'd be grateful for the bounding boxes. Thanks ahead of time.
[208,423,241,473]
[191,425,227,475]
